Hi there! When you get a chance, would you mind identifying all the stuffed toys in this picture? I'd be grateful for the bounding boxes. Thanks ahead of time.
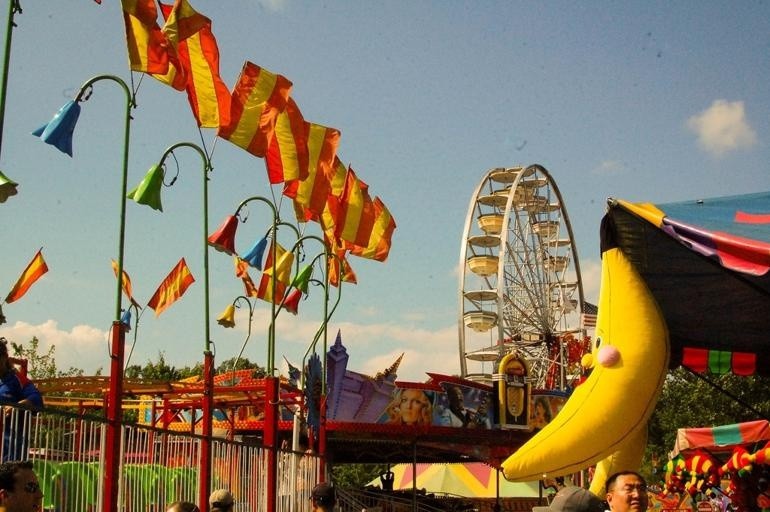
[501,217,671,483]
[656,441,770,499]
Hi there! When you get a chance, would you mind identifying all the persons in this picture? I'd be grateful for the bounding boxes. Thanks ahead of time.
[532,471,648,512]
[756,482,770,512]
[310,484,335,512]
[279,436,318,512]
[167,490,234,512]
[2,341,44,511]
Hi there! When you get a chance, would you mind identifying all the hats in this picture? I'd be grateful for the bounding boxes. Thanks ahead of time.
[531,486,606,512]
[308,482,335,501]
[209,489,235,512]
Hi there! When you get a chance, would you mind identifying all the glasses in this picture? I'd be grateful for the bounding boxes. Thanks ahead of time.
[23,482,40,494]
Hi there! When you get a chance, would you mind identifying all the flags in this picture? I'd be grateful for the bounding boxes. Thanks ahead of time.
[147,258,194,317]
[6,252,49,304]
[217,61,294,159]
[266,98,397,286]
[123,0,233,127]
[584,302,598,328]
[235,242,294,305]
[112,259,142,310]
